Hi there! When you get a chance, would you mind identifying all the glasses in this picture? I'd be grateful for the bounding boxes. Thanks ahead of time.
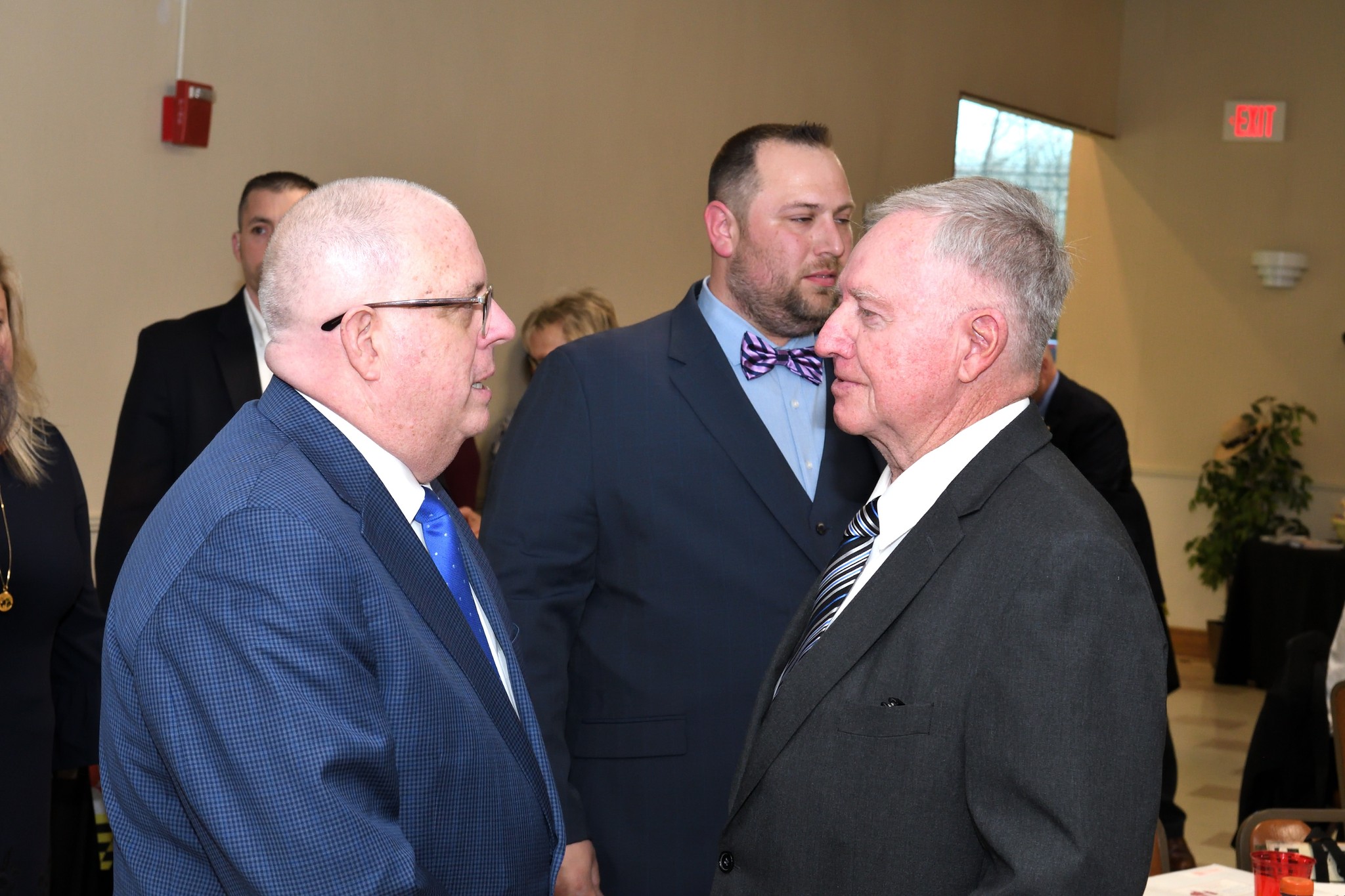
[320,285,493,338]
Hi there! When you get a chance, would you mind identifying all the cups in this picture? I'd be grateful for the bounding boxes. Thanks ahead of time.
[1249,850,1316,896]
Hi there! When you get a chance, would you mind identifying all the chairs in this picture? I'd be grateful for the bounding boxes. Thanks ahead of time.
[1234,804,1345,881]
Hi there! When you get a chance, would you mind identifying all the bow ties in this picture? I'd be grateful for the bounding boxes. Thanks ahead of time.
[740,331,823,386]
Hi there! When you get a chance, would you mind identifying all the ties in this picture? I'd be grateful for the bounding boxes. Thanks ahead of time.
[772,496,881,699]
[414,484,503,686]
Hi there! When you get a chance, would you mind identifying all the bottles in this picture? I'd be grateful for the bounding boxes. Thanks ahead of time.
[1279,876,1314,896]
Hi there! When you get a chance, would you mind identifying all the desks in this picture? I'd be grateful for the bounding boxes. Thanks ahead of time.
[1139,859,1344,896]
[1213,529,1345,689]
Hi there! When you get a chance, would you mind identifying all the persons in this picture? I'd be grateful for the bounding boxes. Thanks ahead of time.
[0,257,99,896]
[95,171,319,603]
[99,176,566,896]
[459,291,618,538]
[1030,345,1196,872]
[479,124,887,896]
[709,175,1169,896]
